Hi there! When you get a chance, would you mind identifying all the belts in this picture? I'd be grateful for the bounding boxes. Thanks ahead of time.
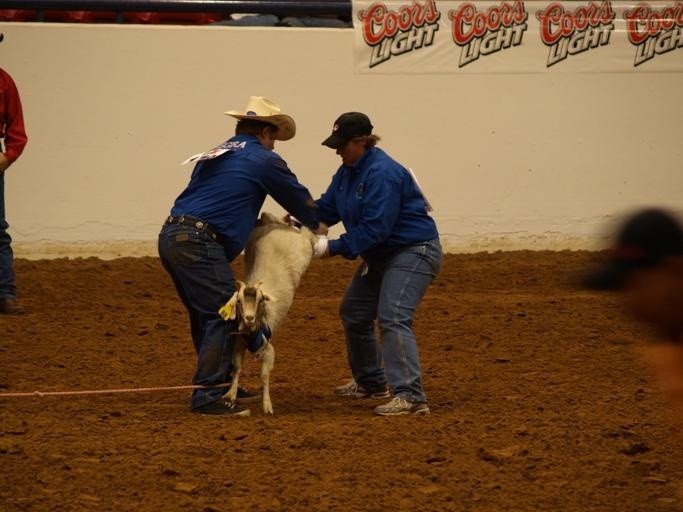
[165,215,224,244]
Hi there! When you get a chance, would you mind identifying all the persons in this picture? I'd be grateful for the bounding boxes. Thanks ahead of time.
[285,112,443,416]
[576,207,683,416]
[0,33,30,314]
[159,98,329,418]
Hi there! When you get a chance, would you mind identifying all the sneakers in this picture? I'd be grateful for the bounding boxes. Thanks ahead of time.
[222,383,262,401]
[192,399,252,418]
[0,297,26,315]
[335,381,391,400]
[373,394,431,416]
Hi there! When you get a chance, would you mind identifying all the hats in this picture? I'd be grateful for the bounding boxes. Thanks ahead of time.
[321,111,371,148]
[580,211,683,292]
[223,94,296,140]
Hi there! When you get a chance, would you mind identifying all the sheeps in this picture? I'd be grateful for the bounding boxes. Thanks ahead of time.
[219,211,320,418]
